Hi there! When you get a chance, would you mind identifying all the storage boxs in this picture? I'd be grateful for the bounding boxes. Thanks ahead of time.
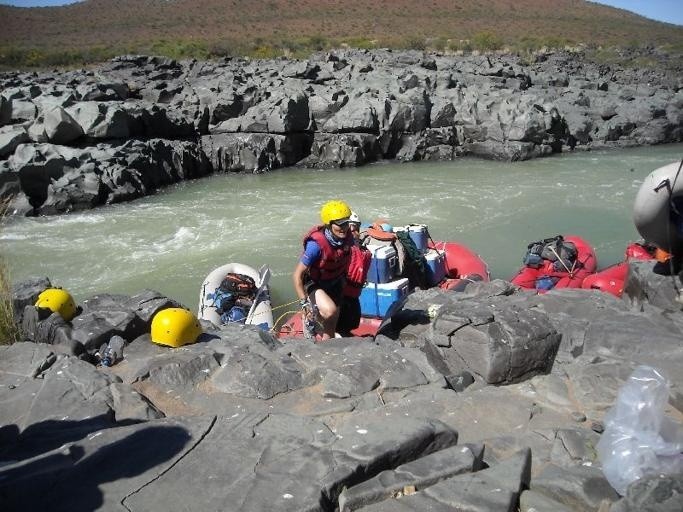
[359,278,409,318]
[409,226,429,254]
[363,245,396,283]
[423,249,446,285]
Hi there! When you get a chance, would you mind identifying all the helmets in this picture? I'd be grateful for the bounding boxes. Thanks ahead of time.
[633,161,683,261]
[348,210,361,226]
[150,306,202,348]
[34,288,76,322]
[321,200,351,227]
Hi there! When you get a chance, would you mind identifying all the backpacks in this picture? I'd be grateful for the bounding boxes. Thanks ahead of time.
[213,272,257,327]
[524,235,578,292]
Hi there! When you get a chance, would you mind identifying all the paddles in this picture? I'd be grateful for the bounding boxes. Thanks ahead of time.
[244,264,270,325]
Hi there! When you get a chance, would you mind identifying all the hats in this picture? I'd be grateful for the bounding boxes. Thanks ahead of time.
[332,219,351,228]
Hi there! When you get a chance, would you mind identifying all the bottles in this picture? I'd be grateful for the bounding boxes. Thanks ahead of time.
[104,344,113,367]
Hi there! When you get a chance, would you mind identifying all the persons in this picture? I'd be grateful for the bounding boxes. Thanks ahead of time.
[344,206,361,242]
[291,198,376,343]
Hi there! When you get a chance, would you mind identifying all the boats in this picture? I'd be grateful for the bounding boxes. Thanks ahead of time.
[198,263,274,333]
[279,239,489,341]
[510,160,683,298]
